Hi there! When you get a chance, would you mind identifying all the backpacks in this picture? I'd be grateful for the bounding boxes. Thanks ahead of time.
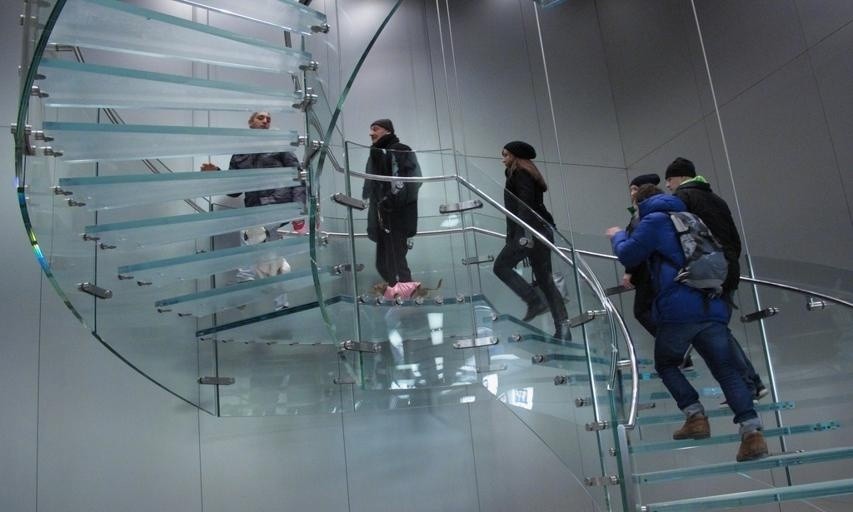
[669,210,730,293]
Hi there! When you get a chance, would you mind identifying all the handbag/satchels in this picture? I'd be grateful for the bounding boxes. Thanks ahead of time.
[528,270,571,315]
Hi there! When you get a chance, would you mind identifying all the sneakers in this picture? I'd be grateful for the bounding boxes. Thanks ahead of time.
[677,353,694,372]
[736,430,769,462]
[674,417,711,439]
[722,374,768,404]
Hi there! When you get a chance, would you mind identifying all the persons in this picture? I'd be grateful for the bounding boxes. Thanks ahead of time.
[362,117,424,299]
[199,110,305,316]
[604,182,768,462]
[664,156,769,407]
[630,173,694,371]
[493,141,573,341]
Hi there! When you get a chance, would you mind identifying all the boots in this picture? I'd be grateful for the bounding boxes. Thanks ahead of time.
[554,318,573,341]
[521,289,547,321]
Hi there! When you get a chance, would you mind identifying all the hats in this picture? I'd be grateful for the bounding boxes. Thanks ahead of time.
[666,156,696,179]
[371,119,394,135]
[505,141,536,160]
[630,173,660,187]
